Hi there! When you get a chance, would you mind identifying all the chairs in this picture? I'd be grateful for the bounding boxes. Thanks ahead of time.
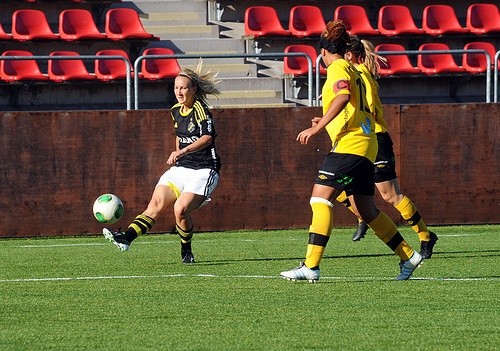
[245,4,500,76]
[0,0,183,81]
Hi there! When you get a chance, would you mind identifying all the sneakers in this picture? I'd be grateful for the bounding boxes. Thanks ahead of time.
[394,251,423,280]
[419,230,438,259]
[181,248,194,263]
[352,221,369,242]
[102,226,130,252]
[280,262,320,283]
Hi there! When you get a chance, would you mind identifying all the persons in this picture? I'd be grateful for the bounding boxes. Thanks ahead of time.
[102,57,221,263]
[279,20,424,283]
[336,40,438,260]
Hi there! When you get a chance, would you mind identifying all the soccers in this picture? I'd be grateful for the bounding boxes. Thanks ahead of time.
[92,193,125,224]
